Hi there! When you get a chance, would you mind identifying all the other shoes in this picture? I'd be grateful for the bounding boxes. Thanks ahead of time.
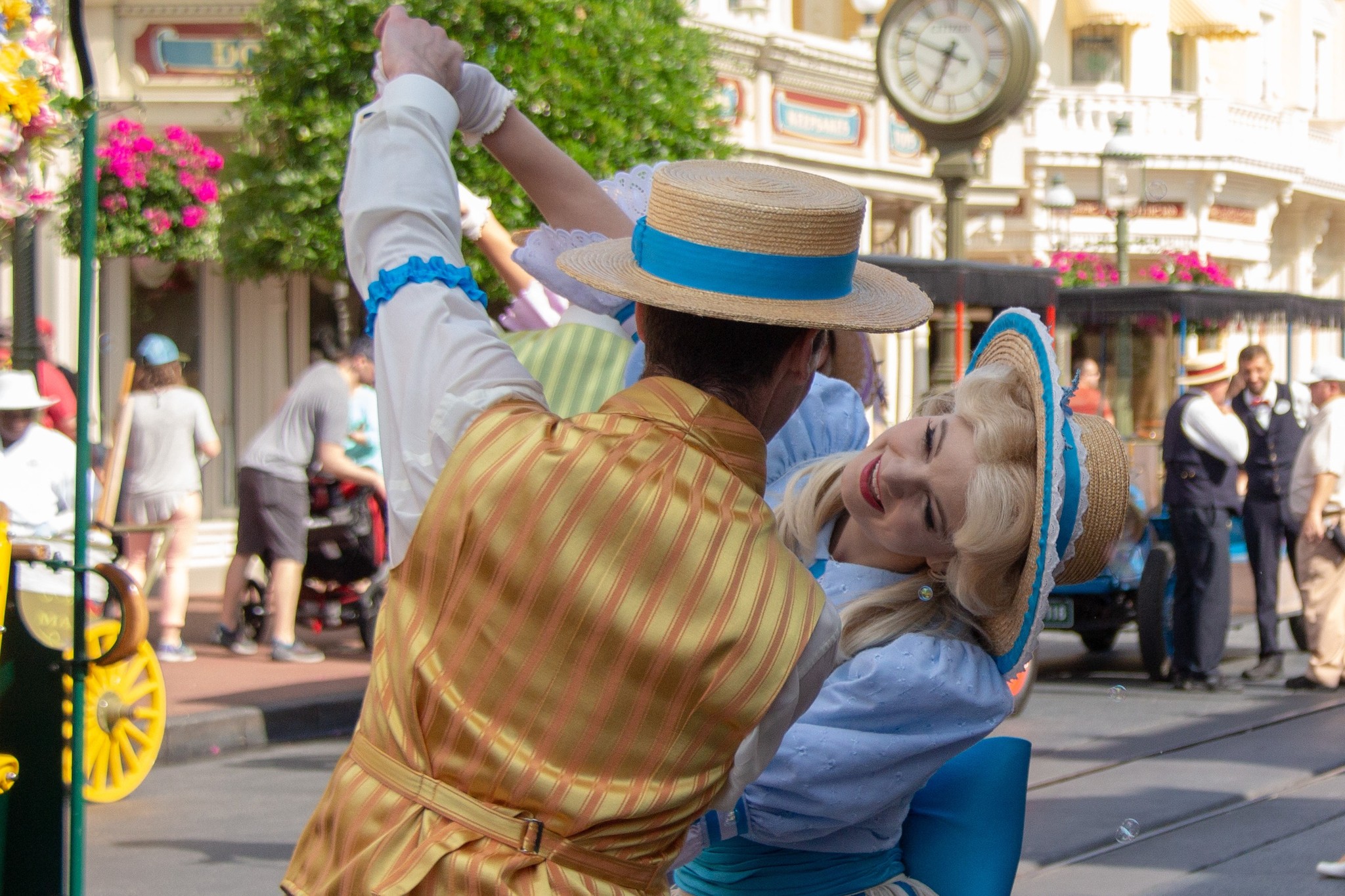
[1242,652,1287,680]
[1174,672,1244,693]
[1317,853,1345,878]
[271,639,326,665]
[211,622,259,655]
[156,641,198,662]
[1285,676,1345,692]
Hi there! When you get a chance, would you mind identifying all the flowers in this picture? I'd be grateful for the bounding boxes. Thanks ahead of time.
[1030,247,1118,325]
[58,119,227,265]
[1138,248,1239,335]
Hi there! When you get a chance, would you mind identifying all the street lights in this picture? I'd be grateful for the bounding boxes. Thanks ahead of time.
[1092,117,1152,432]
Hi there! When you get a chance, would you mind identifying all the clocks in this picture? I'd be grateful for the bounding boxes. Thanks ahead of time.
[874,0,1047,145]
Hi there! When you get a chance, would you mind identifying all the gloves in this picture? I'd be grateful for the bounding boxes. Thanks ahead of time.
[458,179,491,243]
[373,50,517,148]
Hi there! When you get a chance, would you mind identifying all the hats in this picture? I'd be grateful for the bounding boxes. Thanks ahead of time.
[1176,349,1239,386]
[136,333,191,366]
[965,304,1131,683]
[0,370,62,411]
[830,329,892,425]
[557,160,934,332]
[511,228,545,247]
[1302,355,1345,385]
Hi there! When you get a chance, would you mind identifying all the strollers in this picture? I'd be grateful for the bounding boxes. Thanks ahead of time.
[246,477,384,654]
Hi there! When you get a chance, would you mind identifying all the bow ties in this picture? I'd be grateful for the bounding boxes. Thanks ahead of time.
[1250,400,1272,407]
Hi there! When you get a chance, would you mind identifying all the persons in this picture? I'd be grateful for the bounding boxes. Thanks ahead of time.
[1288,357,1345,691]
[1163,352,1248,692]
[1068,356,1115,426]
[372,52,1131,896]
[123,334,221,663]
[280,6,932,896]
[213,337,389,665]
[35,316,77,441]
[0,370,105,537]
[1224,346,1314,684]
